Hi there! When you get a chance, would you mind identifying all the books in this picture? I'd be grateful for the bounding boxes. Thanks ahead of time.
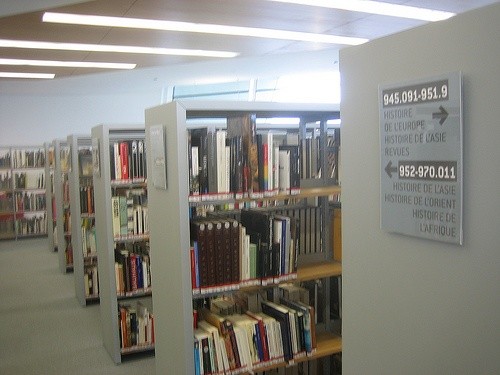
[109,139,148,179]
[119,302,156,347]
[114,241,150,293]
[111,186,148,238]
[1,147,99,299]
[189,111,340,375]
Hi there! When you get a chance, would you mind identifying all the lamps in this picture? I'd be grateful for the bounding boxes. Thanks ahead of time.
[0,0,455,80]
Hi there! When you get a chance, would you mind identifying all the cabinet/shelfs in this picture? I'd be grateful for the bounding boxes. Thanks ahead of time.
[337,0,500,375]
[0,98,341,375]
